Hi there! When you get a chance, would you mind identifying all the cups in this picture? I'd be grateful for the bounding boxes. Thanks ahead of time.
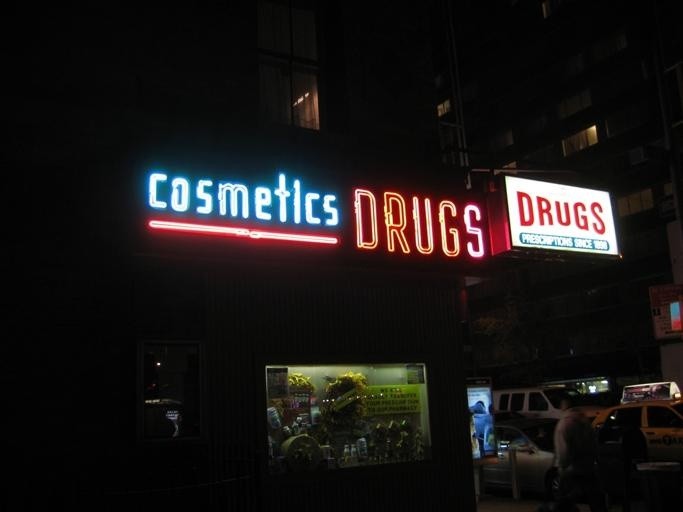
[474,413,491,453]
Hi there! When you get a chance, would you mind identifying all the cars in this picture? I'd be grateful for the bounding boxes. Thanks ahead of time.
[473,382,683,512]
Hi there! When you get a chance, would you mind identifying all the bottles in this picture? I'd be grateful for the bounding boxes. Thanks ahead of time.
[268,437,274,458]
[283,414,307,435]
[483,415,496,453]
[344,444,357,458]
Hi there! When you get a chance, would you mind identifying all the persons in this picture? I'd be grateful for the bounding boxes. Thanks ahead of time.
[549,392,608,512]
